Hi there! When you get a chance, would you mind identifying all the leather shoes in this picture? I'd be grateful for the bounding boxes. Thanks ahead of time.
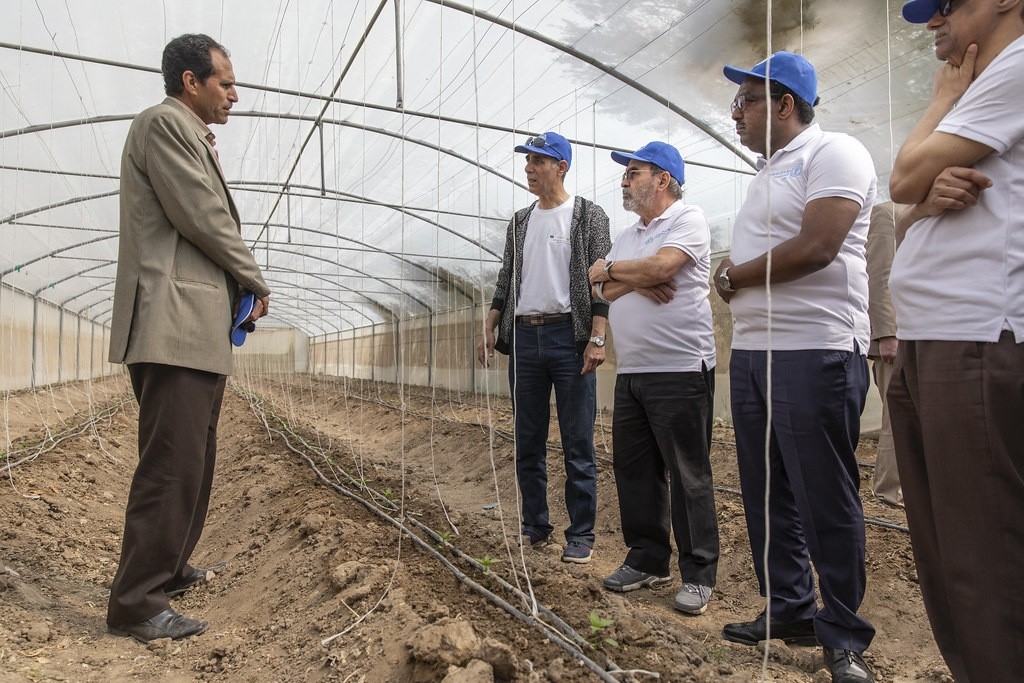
[163,563,226,596]
[722,611,820,646]
[823,644,872,683]
[108,605,208,644]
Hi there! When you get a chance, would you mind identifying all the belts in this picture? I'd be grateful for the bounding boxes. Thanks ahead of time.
[517,315,571,326]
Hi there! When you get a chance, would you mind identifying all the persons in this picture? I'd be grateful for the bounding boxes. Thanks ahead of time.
[478,131,613,564]
[588,140,719,615]
[108,34,271,644]
[865,200,905,508]
[886,0,1024,683]
[712,54,875,683]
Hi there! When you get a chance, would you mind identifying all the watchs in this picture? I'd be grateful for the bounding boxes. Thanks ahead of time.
[589,336,605,346]
[603,260,616,281]
[719,267,737,291]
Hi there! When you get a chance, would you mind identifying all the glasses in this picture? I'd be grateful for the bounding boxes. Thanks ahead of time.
[939,0,954,16]
[732,91,784,114]
[622,169,664,182]
[524,137,564,161]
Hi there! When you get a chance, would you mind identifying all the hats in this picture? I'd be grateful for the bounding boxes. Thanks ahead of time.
[514,131,572,171]
[231,292,253,347]
[723,51,817,107]
[902,0,939,24]
[611,142,685,187]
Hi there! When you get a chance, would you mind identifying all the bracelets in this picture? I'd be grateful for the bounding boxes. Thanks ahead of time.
[596,281,613,303]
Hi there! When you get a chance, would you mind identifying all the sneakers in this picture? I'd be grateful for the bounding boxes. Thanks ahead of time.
[519,530,544,548]
[674,581,712,614]
[602,566,671,592]
[562,541,592,564]
